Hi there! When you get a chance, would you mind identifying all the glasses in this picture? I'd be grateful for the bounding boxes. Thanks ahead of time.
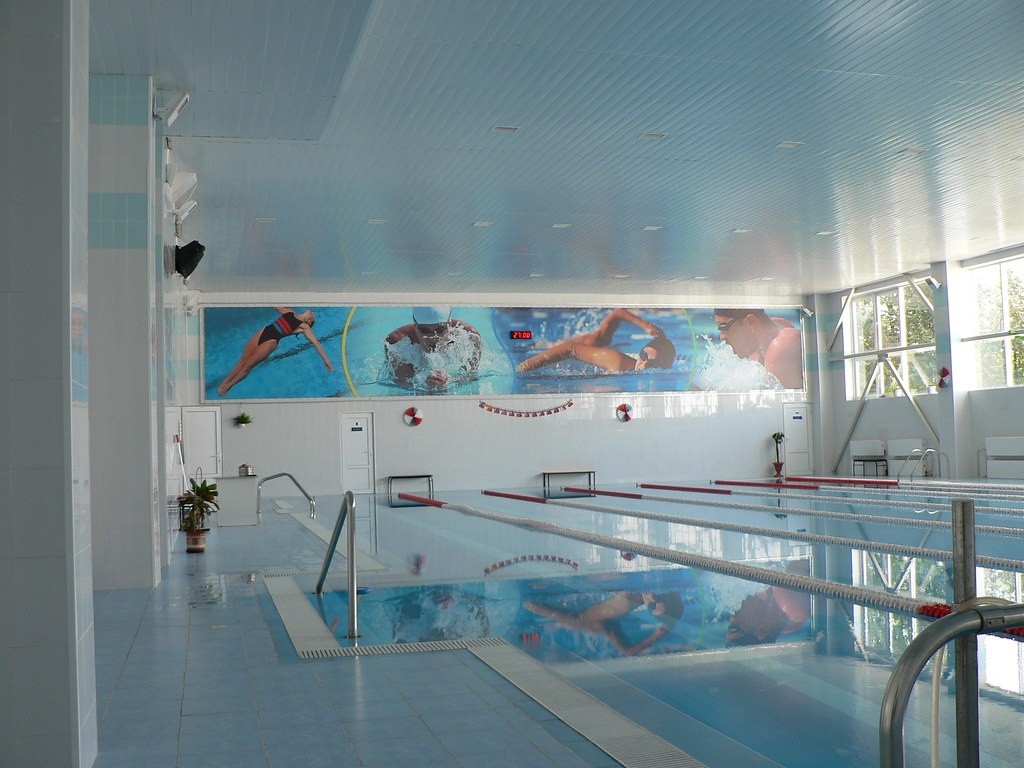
[413,317,450,333]
[718,311,747,332]
[727,624,752,637]
[639,350,657,368]
[648,594,663,611]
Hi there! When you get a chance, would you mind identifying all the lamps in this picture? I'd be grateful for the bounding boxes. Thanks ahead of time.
[153,90,202,317]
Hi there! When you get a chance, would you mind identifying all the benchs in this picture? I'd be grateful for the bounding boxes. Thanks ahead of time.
[387,492,435,508]
[852,459,888,476]
[543,471,596,487]
[543,486,596,499]
[388,475,434,492]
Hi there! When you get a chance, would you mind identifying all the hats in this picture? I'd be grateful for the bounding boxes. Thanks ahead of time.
[413,306,450,324]
[715,308,763,318]
[642,337,676,366]
[655,593,684,624]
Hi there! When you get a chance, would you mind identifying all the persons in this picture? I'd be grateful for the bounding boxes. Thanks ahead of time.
[522,559,810,656]
[216,306,334,397]
[514,308,676,374]
[713,309,803,389]
[383,304,481,392]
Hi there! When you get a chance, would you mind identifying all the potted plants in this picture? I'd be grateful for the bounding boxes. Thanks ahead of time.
[176,479,220,552]
[234,411,254,426]
[772,432,785,476]
[774,479,787,518]
